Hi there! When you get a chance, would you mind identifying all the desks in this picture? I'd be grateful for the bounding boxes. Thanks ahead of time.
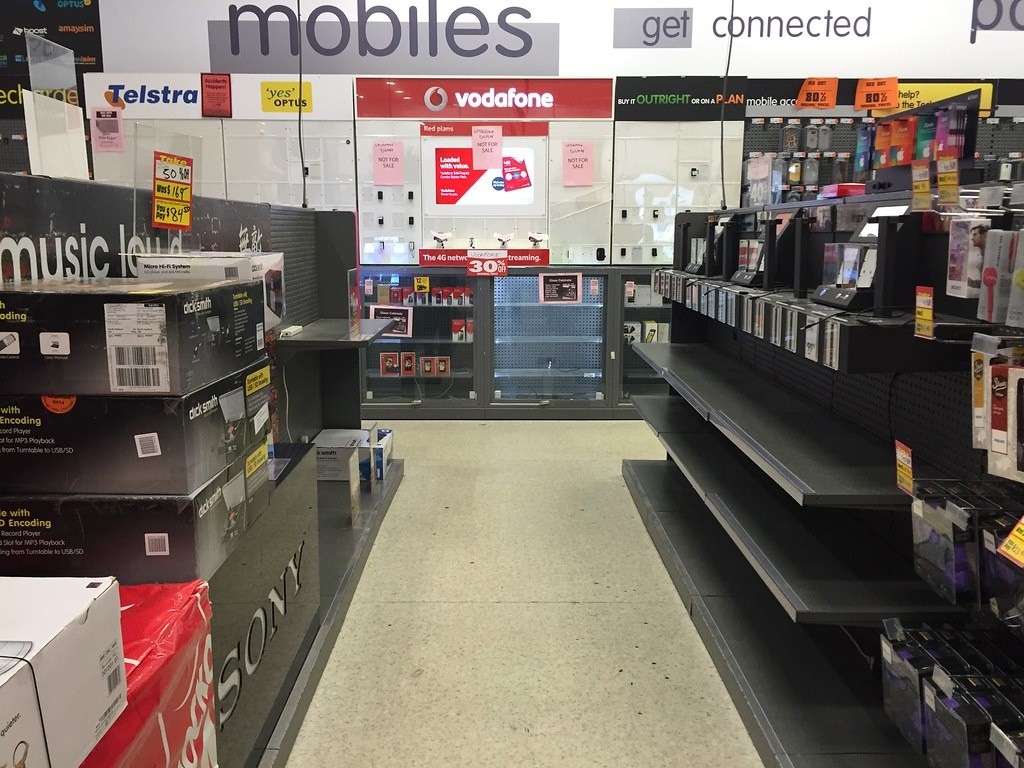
[281,302,388,444]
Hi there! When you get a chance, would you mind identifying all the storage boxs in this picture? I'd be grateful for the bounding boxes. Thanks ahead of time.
[0,252,392,762]
[624,280,670,343]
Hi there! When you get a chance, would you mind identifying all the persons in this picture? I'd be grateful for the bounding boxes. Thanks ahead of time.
[966,225,990,288]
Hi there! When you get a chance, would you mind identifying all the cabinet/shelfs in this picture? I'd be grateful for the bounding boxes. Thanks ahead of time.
[620,261,687,421]
[478,277,617,421]
[354,268,483,413]
[619,185,1024,763]
